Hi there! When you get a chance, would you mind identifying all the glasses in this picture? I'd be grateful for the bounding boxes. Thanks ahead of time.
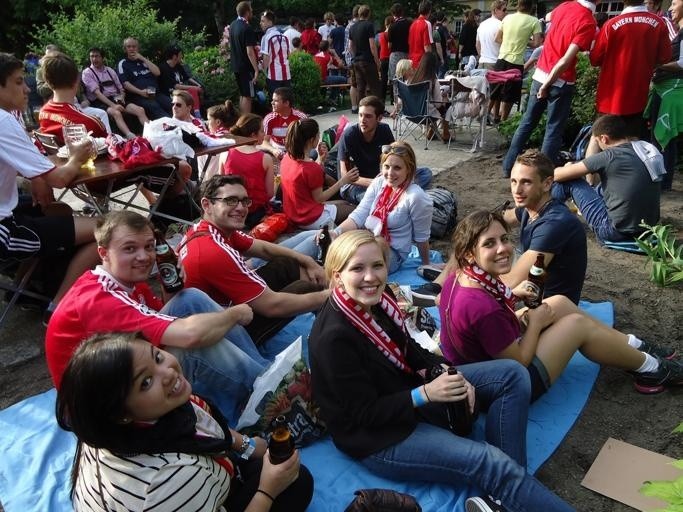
[382,143,407,155]
[171,102,182,108]
[502,7,508,12]
[208,195,254,209]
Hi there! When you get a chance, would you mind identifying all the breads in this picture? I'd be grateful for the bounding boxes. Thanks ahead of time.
[411,306,443,354]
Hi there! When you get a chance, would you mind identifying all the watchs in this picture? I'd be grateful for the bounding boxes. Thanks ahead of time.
[239,433,250,454]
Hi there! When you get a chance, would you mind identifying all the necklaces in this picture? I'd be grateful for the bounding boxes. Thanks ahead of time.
[526,197,553,221]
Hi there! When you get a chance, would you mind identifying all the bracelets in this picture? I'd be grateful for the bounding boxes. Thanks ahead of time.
[423,384,432,403]
[256,489,276,503]
[243,438,256,458]
[412,388,426,407]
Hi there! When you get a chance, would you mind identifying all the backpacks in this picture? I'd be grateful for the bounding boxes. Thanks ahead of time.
[557,124,592,162]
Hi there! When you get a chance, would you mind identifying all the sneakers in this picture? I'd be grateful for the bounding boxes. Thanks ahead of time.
[466,492,508,511]
[632,358,683,395]
[638,339,676,359]
[383,110,391,117]
[416,264,443,282]
[1,285,42,311]
[351,108,358,114]
[443,136,456,144]
[411,283,442,307]
[327,99,337,107]
[428,134,438,140]
[41,309,54,327]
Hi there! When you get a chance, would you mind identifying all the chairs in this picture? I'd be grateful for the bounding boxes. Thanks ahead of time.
[393,77,452,151]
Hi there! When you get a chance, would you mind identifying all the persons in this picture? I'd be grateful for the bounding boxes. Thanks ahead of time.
[408,147,588,320]
[337,96,395,204]
[117,36,172,118]
[229,1,258,114]
[280,116,337,231]
[0,52,108,326]
[585,0,673,187]
[435,13,447,61]
[408,1,434,72]
[282,16,302,53]
[38,51,112,145]
[501,0,603,181]
[160,45,204,122]
[36,44,113,136]
[55,331,314,512]
[308,229,583,512]
[388,3,412,83]
[218,113,274,230]
[348,5,381,101]
[328,13,348,78]
[168,89,207,134]
[343,4,367,114]
[258,10,292,107]
[439,210,683,413]
[317,11,336,40]
[301,18,322,55]
[431,17,445,76]
[291,37,301,53]
[476,0,507,124]
[459,8,482,73]
[197,99,238,175]
[263,86,309,146]
[82,47,150,140]
[643,0,679,43]
[44,209,282,402]
[379,16,396,106]
[644,77,683,192]
[297,22,306,33]
[173,174,332,344]
[408,51,456,144]
[493,0,542,123]
[549,113,661,244]
[652,0,683,75]
[313,40,347,106]
[277,140,432,267]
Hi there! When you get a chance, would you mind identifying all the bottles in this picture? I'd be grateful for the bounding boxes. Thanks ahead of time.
[491,198,511,220]
[343,148,358,173]
[318,220,333,266]
[445,365,474,438]
[150,228,184,295]
[525,251,550,310]
[268,413,296,464]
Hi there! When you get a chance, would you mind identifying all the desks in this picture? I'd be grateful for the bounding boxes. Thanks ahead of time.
[46,126,258,221]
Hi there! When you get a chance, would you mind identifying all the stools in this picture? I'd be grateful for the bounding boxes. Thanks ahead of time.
[321,83,351,108]
[1,254,53,322]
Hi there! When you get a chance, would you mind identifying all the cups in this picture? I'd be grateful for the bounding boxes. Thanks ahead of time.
[63,123,98,169]
